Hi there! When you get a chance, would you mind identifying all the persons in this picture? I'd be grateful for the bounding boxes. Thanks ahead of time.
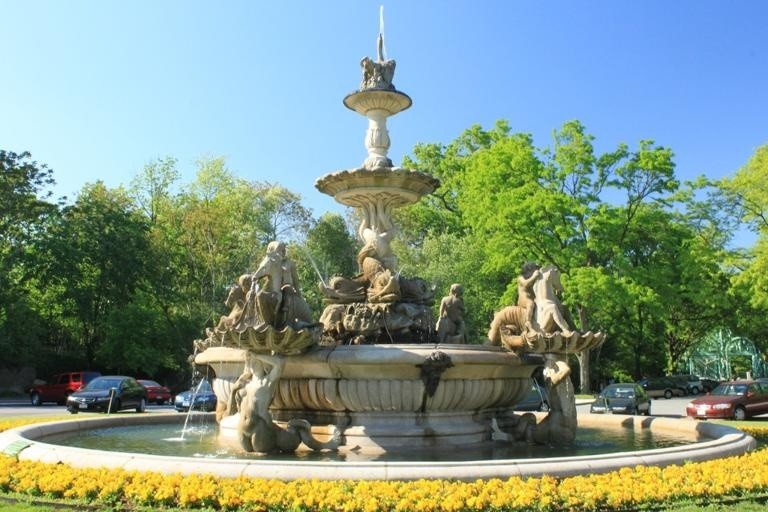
[491,350,579,448]
[438,283,465,334]
[225,353,352,454]
[205,241,300,339]
[518,264,570,336]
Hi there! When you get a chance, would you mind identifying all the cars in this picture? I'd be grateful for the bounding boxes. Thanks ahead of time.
[685,379,768,421]
[174,377,217,413]
[634,374,720,401]
[511,381,550,411]
[135,377,171,405]
[588,381,652,416]
[65,374,148,414]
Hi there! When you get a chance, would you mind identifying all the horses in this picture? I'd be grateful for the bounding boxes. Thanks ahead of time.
[252,251,312,328]
[488,264,579,345]
[217,285,246,331]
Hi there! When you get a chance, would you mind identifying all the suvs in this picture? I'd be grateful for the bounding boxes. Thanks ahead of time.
[23,370,105,407]
